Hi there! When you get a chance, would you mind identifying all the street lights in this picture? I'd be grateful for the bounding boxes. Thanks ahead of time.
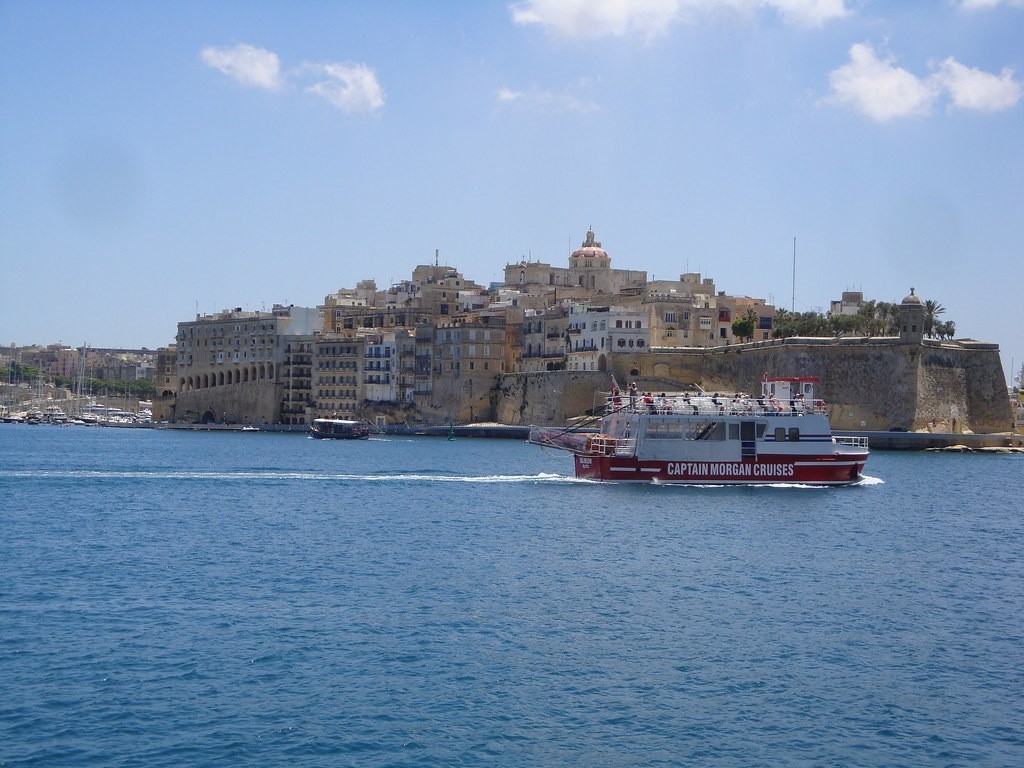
[792,237,795,311]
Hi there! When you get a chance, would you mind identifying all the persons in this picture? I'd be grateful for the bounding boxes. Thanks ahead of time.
[712,393,724,415]
[644,392,672,415]
[624,421,630,438]
[733,393,805,415]
[629,382,637,409]
[613,392,621,405]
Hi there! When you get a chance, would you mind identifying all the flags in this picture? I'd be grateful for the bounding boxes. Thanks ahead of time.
[609,379,617,391]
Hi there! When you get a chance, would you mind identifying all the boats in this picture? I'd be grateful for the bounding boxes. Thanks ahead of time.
[310,411,370,440]
[525,370,870,485]
[241,425,259,433]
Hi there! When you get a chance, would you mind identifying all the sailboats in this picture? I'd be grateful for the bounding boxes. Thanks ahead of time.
[448,422,456,441]
[0,340,153,427]
